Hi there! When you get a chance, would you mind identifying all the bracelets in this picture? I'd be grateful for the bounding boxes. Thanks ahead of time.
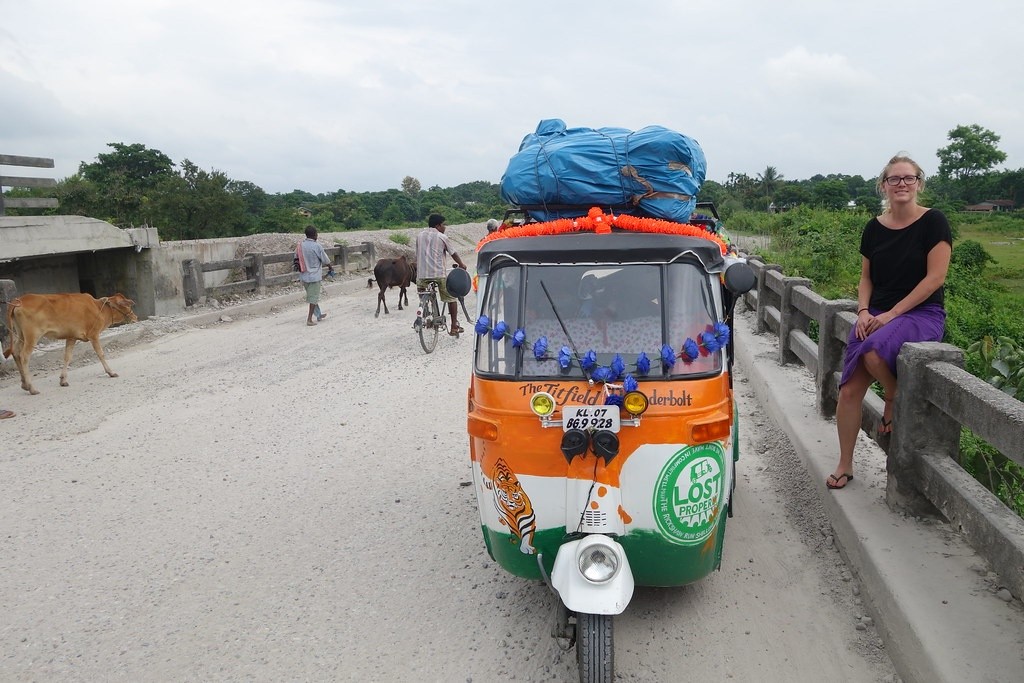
[856,307,870,315]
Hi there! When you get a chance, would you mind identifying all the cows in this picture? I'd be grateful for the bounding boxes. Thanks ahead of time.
[364,255,417,319]
[3,292,139,396]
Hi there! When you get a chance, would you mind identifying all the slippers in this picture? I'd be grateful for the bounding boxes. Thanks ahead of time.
[878,415,893,437]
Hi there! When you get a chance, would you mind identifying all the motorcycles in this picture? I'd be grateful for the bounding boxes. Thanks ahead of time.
[444,199,772,683]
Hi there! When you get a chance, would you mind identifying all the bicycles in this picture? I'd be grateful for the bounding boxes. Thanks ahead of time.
[411,262,467,354]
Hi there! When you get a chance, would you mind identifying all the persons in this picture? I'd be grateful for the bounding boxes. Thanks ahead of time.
[0,340,17,419]
[414,213,468,334]
[293,224,334,326]
[824,151,954,488]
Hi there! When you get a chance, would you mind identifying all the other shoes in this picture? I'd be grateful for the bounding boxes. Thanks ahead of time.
[307,311,325,325]
[451,325,464,334]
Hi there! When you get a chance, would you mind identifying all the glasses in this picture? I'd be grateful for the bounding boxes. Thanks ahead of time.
[827,472,854,488]
[884,175,921,186]
